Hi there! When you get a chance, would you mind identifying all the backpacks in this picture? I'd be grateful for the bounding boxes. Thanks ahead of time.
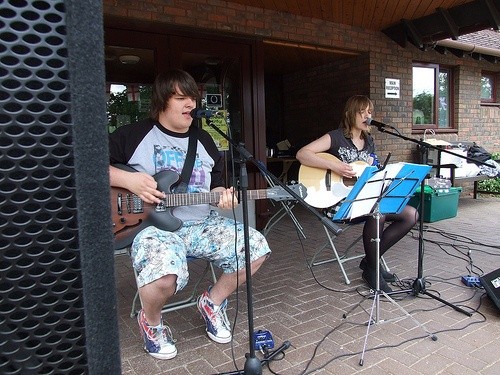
[467,144,492,163]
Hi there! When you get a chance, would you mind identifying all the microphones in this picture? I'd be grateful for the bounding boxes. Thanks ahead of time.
[190,108,222,119]
[366,119,395,129]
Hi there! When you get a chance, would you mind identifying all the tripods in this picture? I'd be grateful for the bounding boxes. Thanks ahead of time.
[201,113,496,375]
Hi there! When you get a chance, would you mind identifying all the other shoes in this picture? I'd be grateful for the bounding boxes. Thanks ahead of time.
[361,267,392,296]
[359,257,396,282]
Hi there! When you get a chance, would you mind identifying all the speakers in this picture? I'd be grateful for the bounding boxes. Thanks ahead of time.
[478,266,500,308]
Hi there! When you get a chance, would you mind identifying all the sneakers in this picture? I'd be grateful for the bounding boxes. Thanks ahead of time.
[196,285,233,344]
[137,309,177,360]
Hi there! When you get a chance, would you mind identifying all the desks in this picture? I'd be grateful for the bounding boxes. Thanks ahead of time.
[266,157,298,185]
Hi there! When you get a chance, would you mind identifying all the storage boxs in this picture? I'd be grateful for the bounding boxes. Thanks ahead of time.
[408,185,463,223]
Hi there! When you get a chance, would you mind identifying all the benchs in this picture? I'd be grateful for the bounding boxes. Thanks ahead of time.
[418,142,500,199]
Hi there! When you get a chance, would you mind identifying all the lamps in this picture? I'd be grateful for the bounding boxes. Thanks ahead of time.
[118,56,142,65]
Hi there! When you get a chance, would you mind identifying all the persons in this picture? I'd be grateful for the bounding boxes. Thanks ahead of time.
[109,67,272,359]
[296,96,419,293]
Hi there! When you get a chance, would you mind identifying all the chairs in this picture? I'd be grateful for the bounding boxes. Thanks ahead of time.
[307,211,390,284]
[257,160,307,240]
[126,243,232,326]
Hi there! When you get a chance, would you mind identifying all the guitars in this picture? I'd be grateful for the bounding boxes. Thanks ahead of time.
[108,162,308,250]
[299,153,430,209]
